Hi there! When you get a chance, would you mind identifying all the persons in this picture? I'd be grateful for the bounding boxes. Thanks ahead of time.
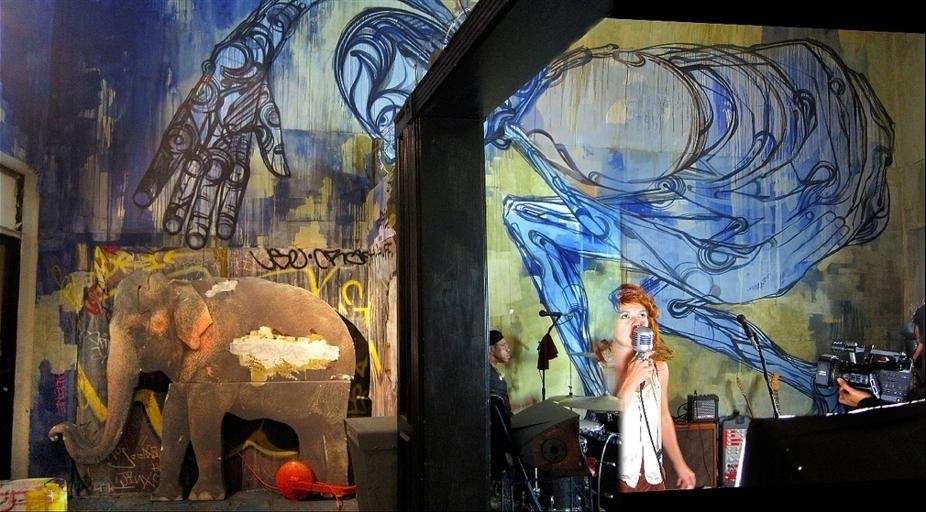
[837,305,926,408]
[490,331,512,415]
[609,283,696,492]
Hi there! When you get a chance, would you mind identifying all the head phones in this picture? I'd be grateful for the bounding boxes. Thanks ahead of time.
[275,463,356,500]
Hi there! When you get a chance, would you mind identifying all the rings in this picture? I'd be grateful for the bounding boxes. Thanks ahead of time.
[647,367,652,371]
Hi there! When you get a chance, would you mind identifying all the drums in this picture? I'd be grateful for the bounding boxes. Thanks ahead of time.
[579,428,620,493]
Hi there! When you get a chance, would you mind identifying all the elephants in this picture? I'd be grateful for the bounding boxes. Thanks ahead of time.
[48,270,355,502]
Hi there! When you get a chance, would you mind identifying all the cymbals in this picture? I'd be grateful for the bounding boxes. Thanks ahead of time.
[548,396,585,402]
[559,395,624,411]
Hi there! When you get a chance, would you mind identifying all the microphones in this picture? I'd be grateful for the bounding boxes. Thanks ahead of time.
[632,326,654,389]
[539,310,573,318]
[736,314,760,352]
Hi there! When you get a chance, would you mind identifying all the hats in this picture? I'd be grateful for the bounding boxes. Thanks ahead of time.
[489,331,503,345]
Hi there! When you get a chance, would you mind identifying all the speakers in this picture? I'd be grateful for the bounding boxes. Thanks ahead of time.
[511,401,578,479]
